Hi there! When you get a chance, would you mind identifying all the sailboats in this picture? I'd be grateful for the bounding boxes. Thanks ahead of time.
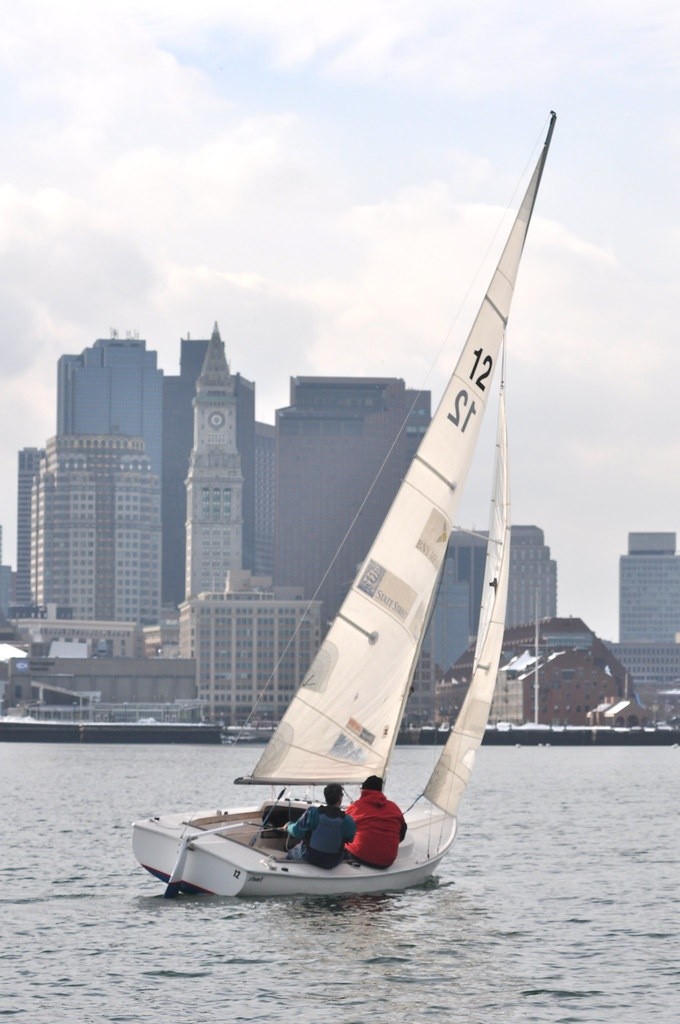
[128,111,559,901]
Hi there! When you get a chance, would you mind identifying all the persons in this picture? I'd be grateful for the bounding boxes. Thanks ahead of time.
[283,784,356,869]
[344,776,406,869]
